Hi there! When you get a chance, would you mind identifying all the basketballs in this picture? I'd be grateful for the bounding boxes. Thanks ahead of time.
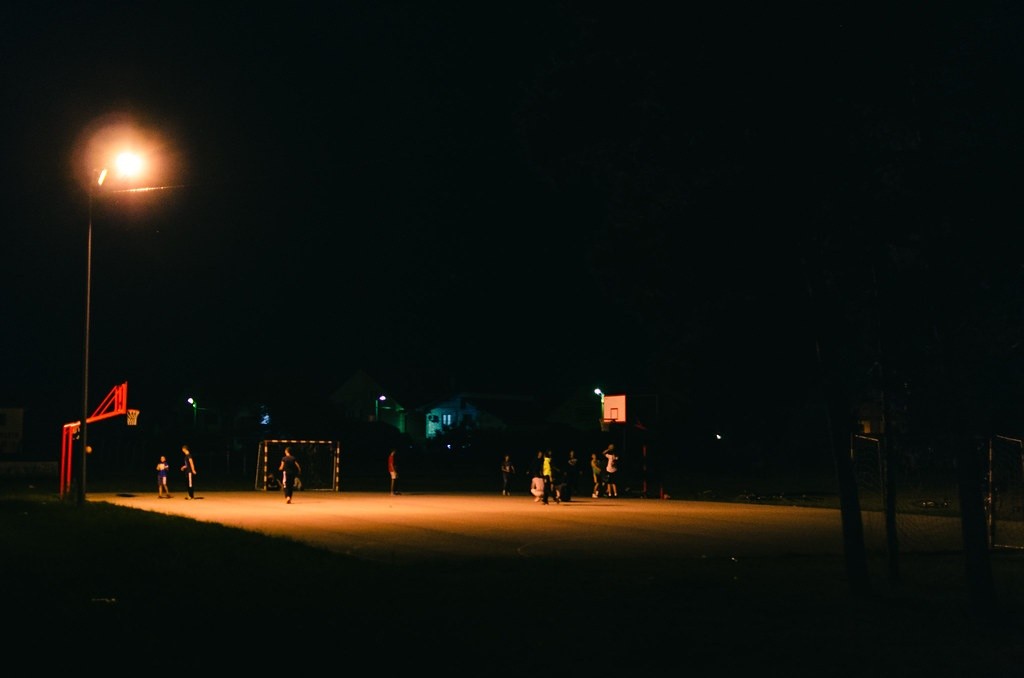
[608,444,614,449]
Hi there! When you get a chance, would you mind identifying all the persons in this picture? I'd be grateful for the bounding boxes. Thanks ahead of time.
[279,447,301,504]
[156,456,170,498]
[267,473,281,491]
[293,477,303,491]
[603,449,619,497]
[181,445,197,500]
[501,456,514,496]
[534,450,578,505]
[591,453,602,498]
[388,448,398,495]
